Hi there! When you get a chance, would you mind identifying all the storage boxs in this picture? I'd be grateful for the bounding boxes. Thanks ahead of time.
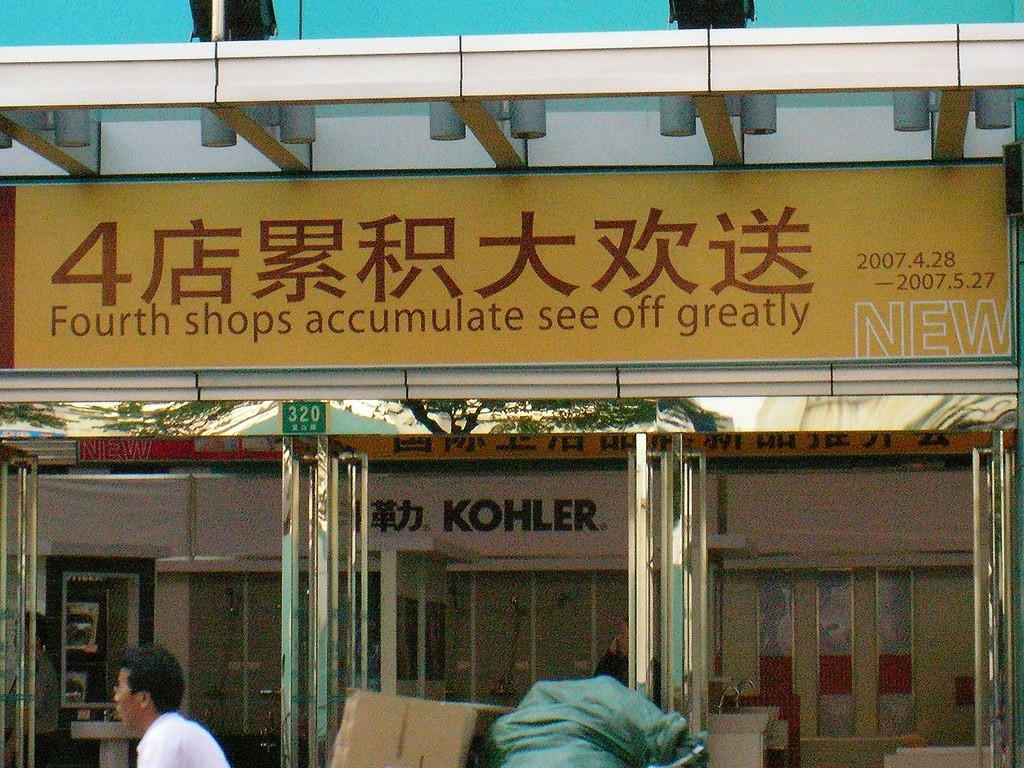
[326,687,517,768]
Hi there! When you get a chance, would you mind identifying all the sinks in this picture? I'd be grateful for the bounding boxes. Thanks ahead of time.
[709,704,780,734]
[70,721,137,740]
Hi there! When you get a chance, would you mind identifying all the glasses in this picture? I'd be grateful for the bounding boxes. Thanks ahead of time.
[113,686,138,695]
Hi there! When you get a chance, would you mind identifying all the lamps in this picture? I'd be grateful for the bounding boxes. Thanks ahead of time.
[189,0,277,42]
[668,0,754,29]
[1,85,1012,148]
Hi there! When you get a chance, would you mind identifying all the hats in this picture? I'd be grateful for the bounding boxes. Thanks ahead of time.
[15,610,49,645]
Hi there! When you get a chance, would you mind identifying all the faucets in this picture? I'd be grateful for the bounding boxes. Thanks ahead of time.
[734,679,756,707]
[717,684,739,715]
[103,709,117,722]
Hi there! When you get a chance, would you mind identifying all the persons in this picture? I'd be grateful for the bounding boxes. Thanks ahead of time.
[593,616,661,710]
[6,612,58,768]
[113,642,231,768]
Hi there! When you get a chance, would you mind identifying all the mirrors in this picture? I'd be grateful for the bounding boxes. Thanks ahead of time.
[59,571,140,710]
